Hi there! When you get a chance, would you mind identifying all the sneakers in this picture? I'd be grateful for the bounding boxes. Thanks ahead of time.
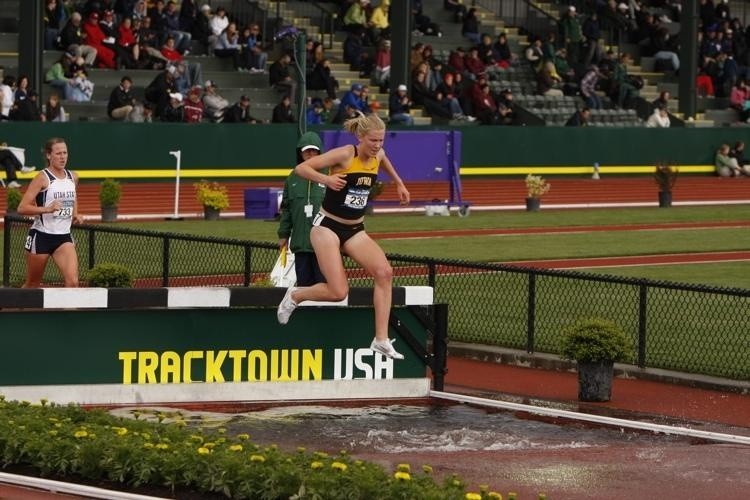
[371,336,404,360]
[277,289,299,325]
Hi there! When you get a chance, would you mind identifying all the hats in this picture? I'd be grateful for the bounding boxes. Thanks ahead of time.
[300,145,319,153]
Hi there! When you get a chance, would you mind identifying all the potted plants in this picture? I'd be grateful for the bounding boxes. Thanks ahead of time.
[653,161,680,208]
[98,178,121,222]
[7,187,24,227]
[194,180,230,221]
[524,173,552,211]
[558,317,635,403]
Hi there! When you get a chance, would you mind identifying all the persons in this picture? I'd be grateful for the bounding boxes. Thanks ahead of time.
[17,137,83,311]
[277,110,410,360]
[278,132,329,286]
[1,1,376,188]
[341,1,750,129]
[715,140,750,177]
